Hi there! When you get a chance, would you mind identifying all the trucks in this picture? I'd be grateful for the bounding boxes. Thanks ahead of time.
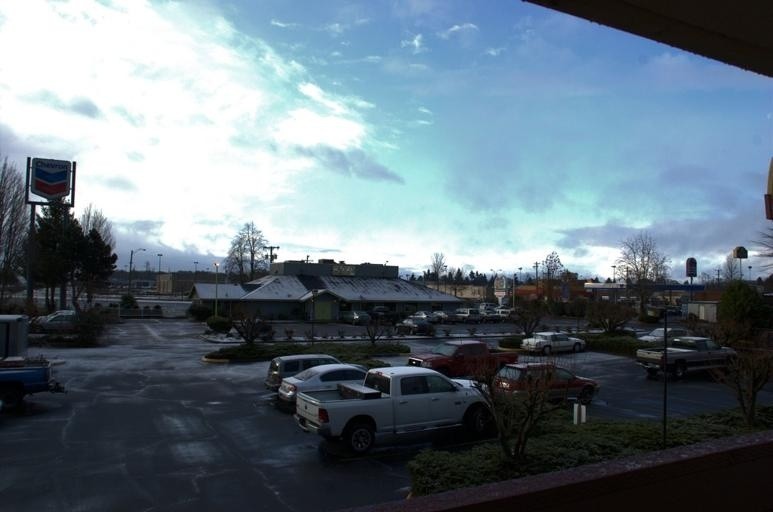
[0,314,29,358]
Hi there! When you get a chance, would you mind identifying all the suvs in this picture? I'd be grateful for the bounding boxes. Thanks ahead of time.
[490,361,601,406]
[30,309,82,335]
[450,307,480,323]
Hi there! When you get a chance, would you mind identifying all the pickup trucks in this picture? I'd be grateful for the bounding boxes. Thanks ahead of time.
[1,355,56,404]
[290,366,498,454]
[634,335,738,380]
[407,338,519,378]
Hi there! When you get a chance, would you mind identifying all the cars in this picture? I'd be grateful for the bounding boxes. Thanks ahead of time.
[369,306,394,321]
[477,309,499,323]
[335,309,370,325]
[407,309,457,325]
[393,317,434,337]
[518,330,587,357]
[634,326,697,350]
[275,362,371,405]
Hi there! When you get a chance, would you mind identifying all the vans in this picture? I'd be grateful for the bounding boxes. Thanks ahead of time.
[479,302,496,311]
[494,308,513,322]
[263,353,344,393]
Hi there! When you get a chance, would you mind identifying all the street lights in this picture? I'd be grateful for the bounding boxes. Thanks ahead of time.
[518,267,521,282]
[213,260,219,316]
[489,268,494,296]
[611,265,615,280]
[309,287,318,345]
[157,253,163,299]
[653,296,681,449]
[193,261,198,282]
[493,269,502,295]
[127,248,145,297]
